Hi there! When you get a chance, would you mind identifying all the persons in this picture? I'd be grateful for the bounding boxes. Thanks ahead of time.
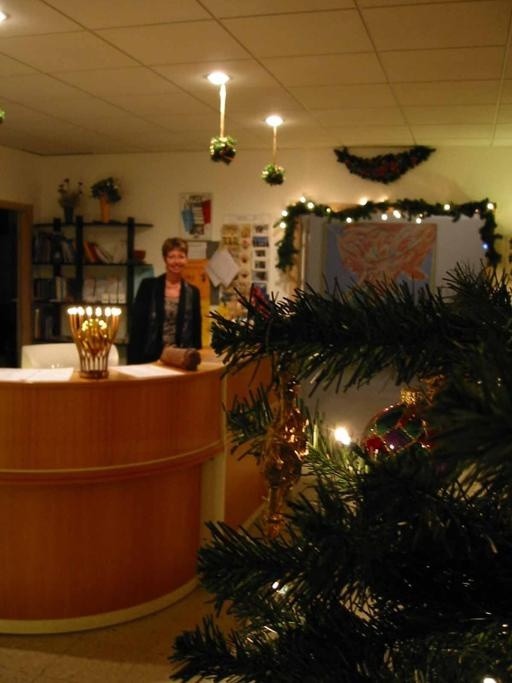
[126,236,202,365]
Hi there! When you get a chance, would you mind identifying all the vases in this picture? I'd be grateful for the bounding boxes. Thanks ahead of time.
[63,207,74,222]
[99,195,111,221]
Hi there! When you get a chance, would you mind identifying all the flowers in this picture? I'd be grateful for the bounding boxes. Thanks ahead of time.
[56,178,84,207]
[261,163,285,185]
[334,145,437,184]
[209,135,238,165]
[90,176,122,203]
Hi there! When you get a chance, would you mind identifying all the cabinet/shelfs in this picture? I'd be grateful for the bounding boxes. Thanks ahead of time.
[32,220,152,344]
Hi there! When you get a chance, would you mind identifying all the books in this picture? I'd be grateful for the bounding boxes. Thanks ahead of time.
[30,231,114,341]
